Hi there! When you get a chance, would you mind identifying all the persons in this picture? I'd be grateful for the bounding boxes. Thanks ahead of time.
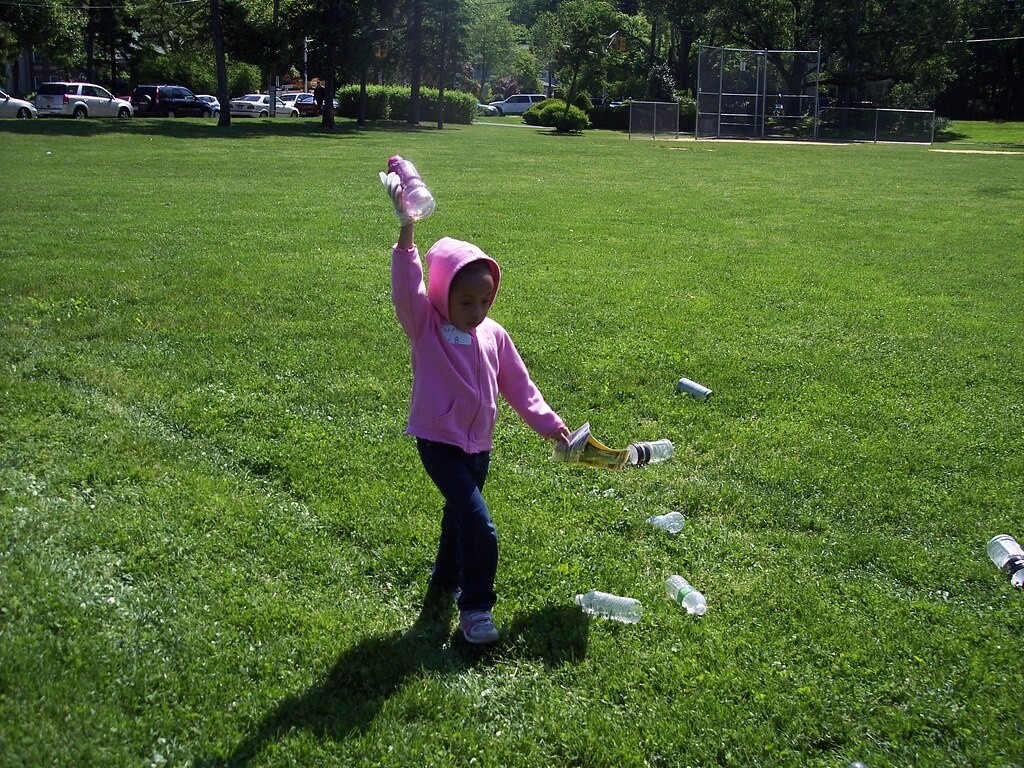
[378,171,572,645]
[313,82,324,115]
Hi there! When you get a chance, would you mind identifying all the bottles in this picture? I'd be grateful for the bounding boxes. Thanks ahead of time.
[986,534,1024,588]
[388,154,435,221]
[645,512,685,535]
[665,576,707,616]
[848,763,865,767]
[628,439,673,465]
[575,591,643,625]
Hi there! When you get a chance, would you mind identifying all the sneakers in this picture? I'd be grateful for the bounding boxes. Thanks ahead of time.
[423,577,462,608]
[459,608,499,644]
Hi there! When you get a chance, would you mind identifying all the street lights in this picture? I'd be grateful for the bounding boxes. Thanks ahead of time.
[753,52,764,132]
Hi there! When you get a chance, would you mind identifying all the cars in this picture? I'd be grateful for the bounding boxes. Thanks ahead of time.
[477,100,498,117]
[230,94,300,119]
[0,91,38,120]
[294,93,339,117]
[277,93,314,107]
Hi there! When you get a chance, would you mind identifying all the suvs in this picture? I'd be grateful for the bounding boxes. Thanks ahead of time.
[489,94,547,117]
[131,83,213,119]
[184,95,220,118]
[36,82,134,120]
